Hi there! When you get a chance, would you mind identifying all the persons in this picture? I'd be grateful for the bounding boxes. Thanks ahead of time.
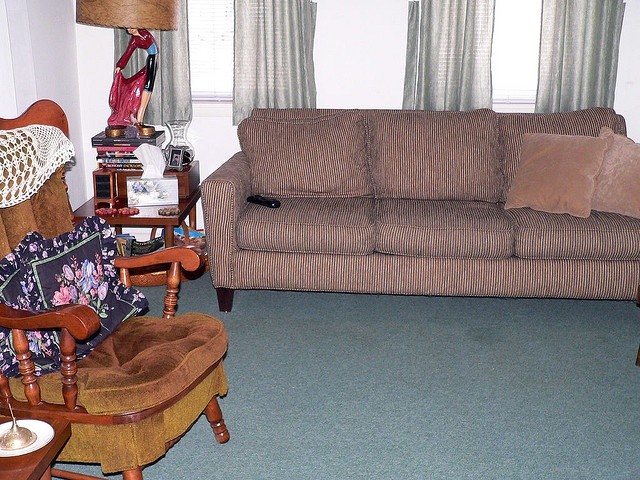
[115,28,159,126]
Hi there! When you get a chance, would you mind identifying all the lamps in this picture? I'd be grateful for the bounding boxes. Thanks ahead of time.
[75,0,181,139]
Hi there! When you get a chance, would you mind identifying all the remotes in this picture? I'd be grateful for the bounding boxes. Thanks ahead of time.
[246,195,281,208]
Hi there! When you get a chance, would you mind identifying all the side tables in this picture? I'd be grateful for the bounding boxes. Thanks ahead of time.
[0,409,73,480]
[70,183,210,288]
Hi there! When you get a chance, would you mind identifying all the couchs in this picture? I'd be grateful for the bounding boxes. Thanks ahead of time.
[195,106,640,314]
[0,98,231,480]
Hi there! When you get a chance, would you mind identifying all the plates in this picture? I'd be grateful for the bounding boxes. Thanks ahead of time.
[0,419,54,459]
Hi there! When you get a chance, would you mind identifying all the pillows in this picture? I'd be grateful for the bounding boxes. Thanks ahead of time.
[592,125,640,220]
[502,130,616,220]
[0,230,61,376]
[12,215,150,367]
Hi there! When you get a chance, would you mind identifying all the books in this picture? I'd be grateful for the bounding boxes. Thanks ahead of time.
[91,130,166,171]
